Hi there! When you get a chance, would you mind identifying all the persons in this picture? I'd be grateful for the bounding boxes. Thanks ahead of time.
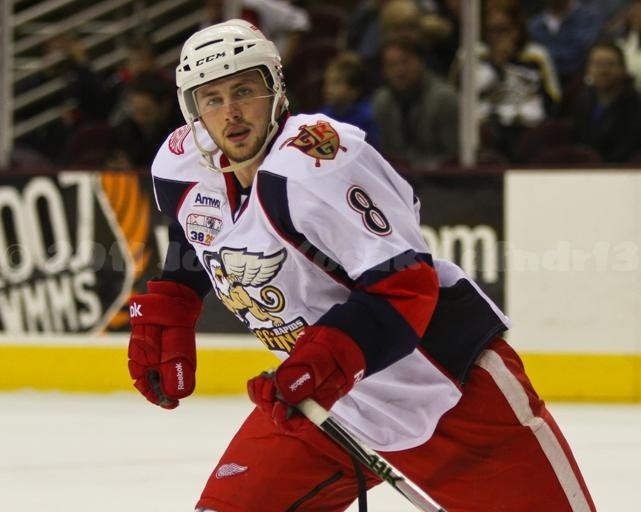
[1,0,641,181]
[126,17,602,511]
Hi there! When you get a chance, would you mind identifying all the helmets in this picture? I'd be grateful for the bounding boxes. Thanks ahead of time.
[176,19,290,172]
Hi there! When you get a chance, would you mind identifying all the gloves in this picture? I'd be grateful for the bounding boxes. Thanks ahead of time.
[128,278,205,408]
[246,326,368,439]
